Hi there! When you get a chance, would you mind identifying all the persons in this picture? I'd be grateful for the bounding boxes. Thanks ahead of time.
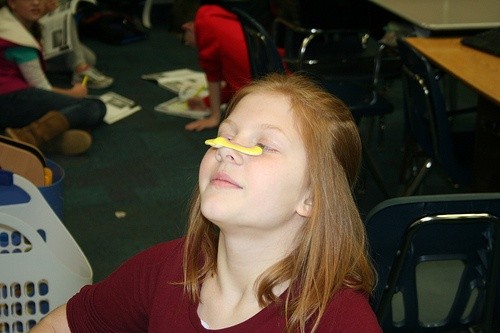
[0,0,292,155]
[31,74,382,333]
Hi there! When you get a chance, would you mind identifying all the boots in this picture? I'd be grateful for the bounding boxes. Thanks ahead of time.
[38,128,94,158]
[4,109,71,148]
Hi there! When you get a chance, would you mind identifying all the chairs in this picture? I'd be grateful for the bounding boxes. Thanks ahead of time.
[234,9,500,333]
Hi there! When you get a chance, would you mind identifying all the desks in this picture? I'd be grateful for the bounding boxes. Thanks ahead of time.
[372,0,500,31]
[402,37,500,106]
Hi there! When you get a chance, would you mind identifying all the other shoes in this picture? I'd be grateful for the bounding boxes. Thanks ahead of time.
[71,64,114,90]
